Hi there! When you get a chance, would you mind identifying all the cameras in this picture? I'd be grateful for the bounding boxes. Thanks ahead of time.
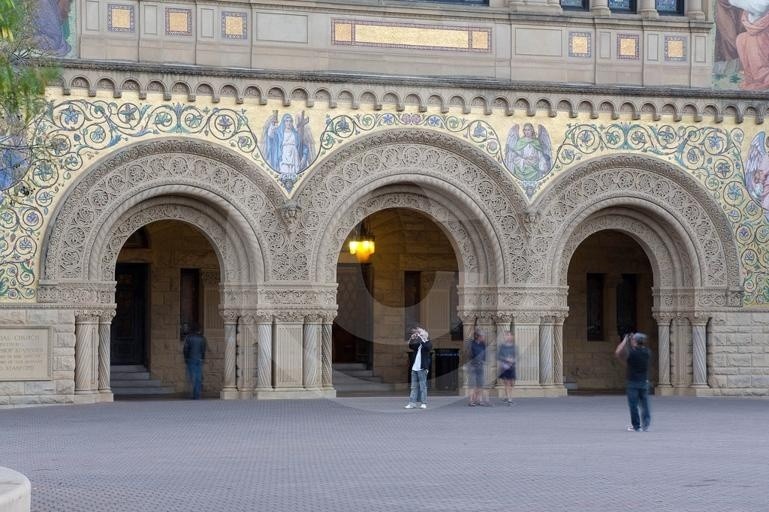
[415,333,419,337]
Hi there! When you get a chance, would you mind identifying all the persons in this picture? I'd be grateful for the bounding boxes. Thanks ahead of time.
[183,325,207,399]
[755,138,769,209]
[404,328,432,409]
[463,329,491,406]
[510,123,548,180]
[614,332,652,433]
[268,113,308,173]
[494,331,520,404]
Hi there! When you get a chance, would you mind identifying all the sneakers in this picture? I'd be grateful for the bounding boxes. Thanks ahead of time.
[627,426,648,432]
[405,403,426,409]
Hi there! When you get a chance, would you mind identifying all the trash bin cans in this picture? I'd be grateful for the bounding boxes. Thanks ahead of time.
[407,348,460,396]
